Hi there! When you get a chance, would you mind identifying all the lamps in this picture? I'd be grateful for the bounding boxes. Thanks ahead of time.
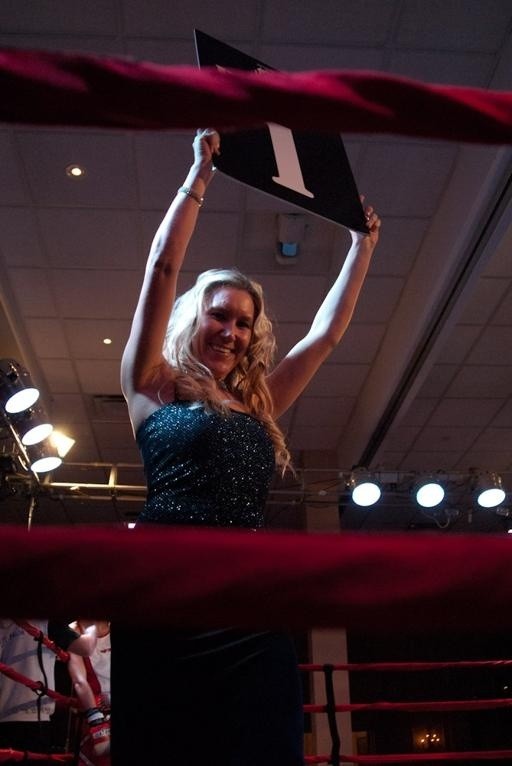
[0,356,76,476]
[339,468,506,508]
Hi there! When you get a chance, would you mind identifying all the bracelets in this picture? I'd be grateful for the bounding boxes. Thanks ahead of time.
[178,186,205,208]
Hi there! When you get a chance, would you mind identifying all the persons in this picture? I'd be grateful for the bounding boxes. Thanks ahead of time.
[66,620,112,766]
[0,617,97,766]
[111,127,383,766]
